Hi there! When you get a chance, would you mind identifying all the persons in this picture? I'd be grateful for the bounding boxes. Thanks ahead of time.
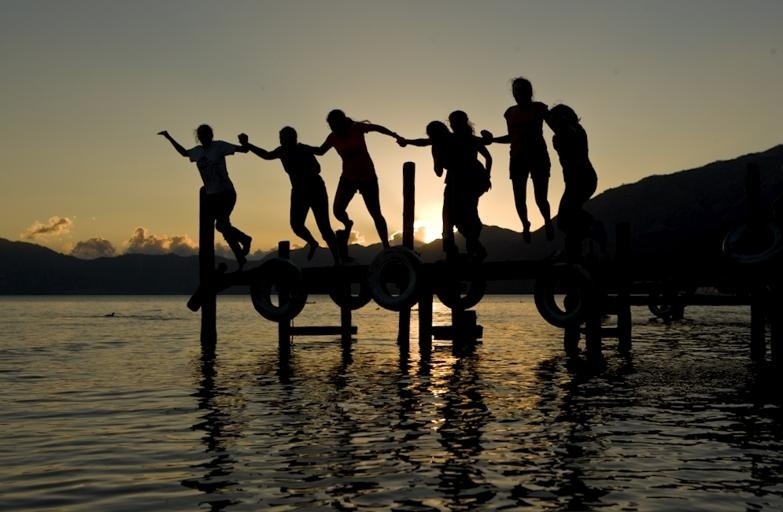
[397,110,493,256]
[240,126,341,260]
[319,110,407,254]
[158,125,251,271]
[481,78,556,244]
[550,104,606,256]
[427,121,492,263]
